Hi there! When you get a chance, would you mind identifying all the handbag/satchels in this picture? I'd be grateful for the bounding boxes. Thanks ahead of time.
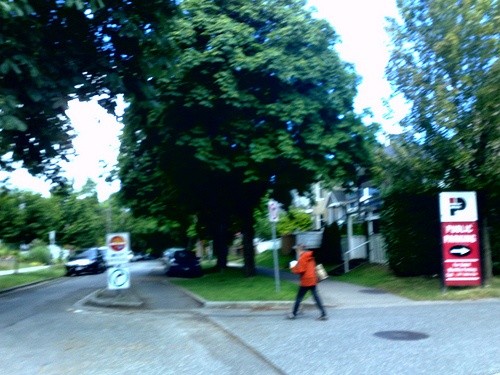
[315,264,328,280]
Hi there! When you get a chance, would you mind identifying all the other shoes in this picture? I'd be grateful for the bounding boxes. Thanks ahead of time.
[289,313,295,318]
[317,316,328,320]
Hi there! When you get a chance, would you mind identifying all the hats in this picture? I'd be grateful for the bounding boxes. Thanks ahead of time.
[292,244,306,249]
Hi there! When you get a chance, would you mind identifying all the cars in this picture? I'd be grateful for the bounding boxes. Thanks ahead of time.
[65,247,105,276]
[160,247,204,277]
